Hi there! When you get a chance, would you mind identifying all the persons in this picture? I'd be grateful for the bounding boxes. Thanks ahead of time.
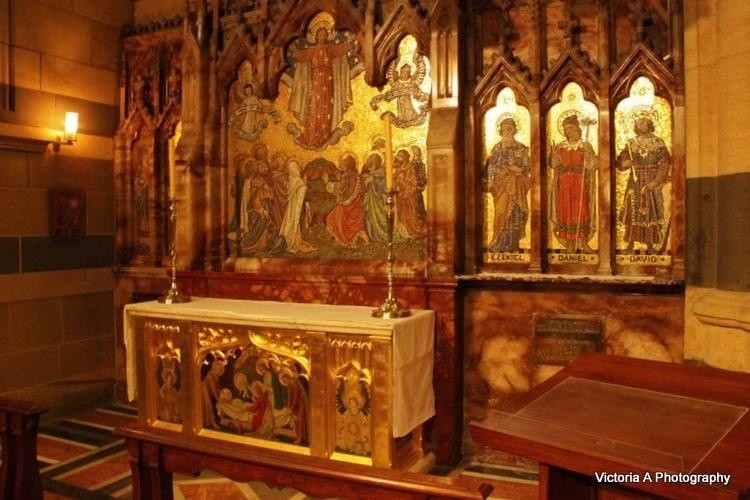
[480,111,531,249]
[230,146,430,253]
[201,351,309,446]
[547,114,600,255]
[290,25,358,151]
[157,367,178,423]
[613,105,673,255]
[390,63,420,121]
[237,82,264,135]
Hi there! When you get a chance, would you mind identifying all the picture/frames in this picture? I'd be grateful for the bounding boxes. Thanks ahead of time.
[48,184,86,242]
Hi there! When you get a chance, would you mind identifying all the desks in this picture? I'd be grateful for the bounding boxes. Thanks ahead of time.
[469,354,750,500]
[123,295,436,475]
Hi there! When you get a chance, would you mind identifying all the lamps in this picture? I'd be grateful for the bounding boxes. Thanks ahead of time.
[53,111,79,152]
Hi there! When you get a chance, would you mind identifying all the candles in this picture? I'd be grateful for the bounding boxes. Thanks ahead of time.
[167,138,177,198]
[383,112,395,192]
